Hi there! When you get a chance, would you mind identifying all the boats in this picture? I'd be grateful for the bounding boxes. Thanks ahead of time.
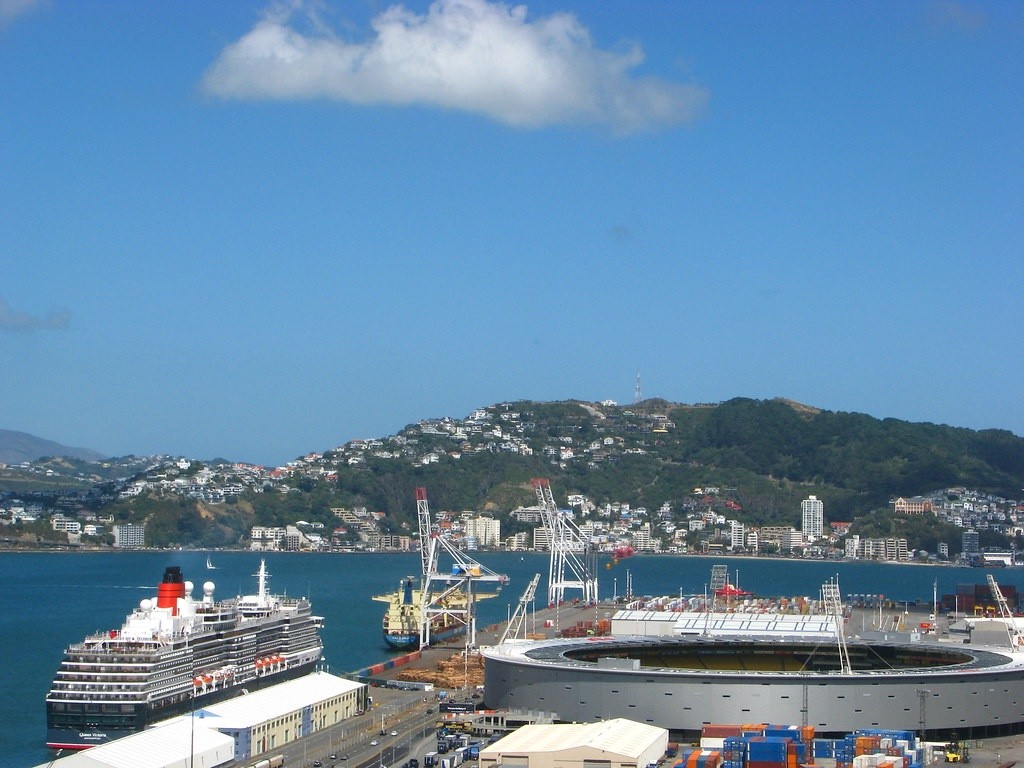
[713,582,754,599]
[44,558,326,729]
[371,577,499,651]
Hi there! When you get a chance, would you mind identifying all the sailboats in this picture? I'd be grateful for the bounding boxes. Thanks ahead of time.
[207,554,218,570]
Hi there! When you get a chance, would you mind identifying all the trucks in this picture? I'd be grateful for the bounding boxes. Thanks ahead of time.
[404,730,479,768]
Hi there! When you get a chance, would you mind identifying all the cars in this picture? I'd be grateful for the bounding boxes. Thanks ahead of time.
[370,739,379,746]
[341,754,349,760]
[391,730,398,736]
[314,761,322,767]
[330,753,337,759]
[372,682,419,692]
